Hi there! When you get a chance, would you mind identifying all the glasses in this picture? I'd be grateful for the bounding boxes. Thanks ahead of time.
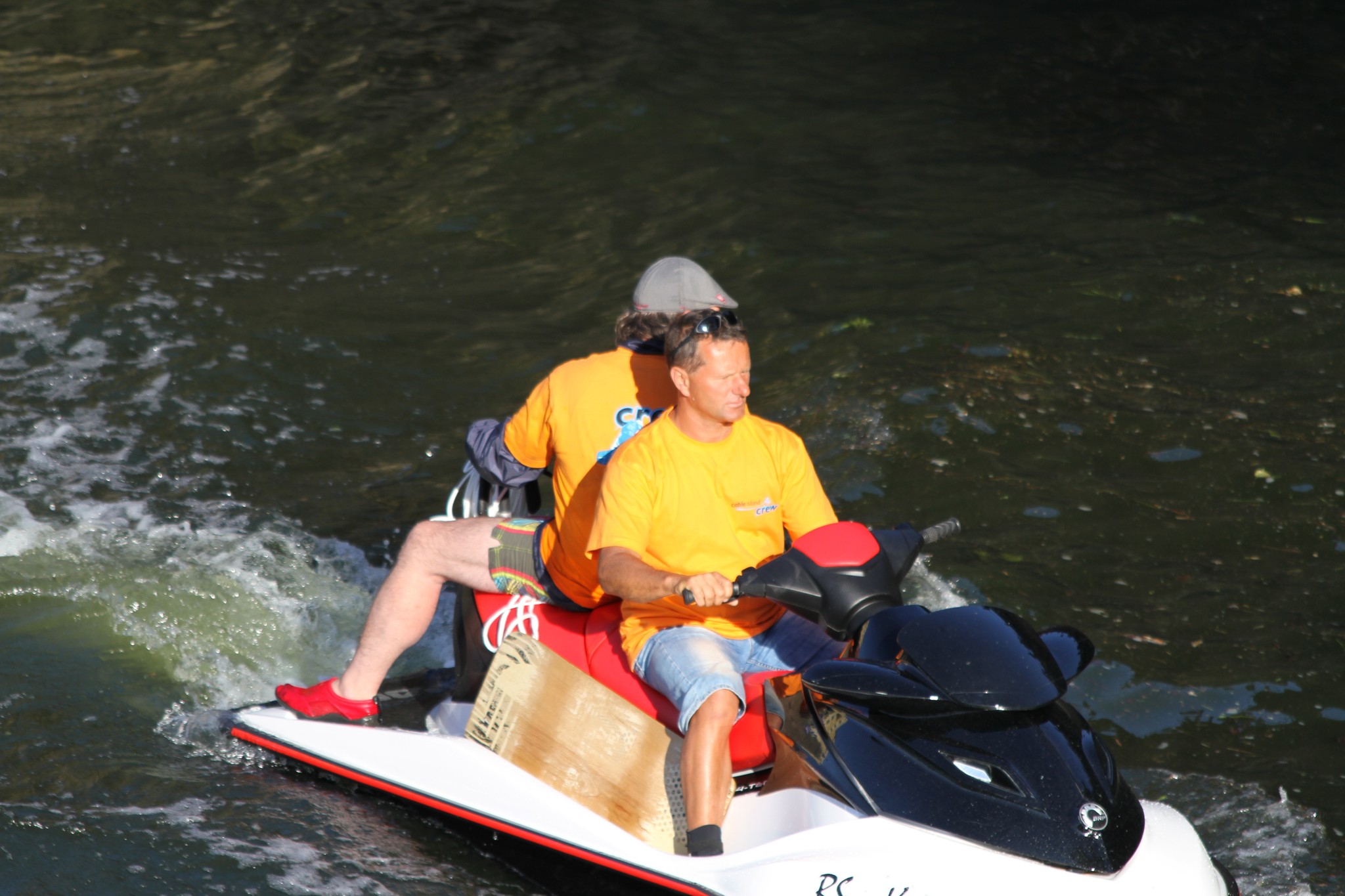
[671,309,738,359]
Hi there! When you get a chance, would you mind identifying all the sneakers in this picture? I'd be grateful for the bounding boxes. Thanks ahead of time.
[274,677,380,727]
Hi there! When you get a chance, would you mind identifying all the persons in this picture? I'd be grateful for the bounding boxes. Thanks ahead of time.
[582,310,842,857]
[275,255,779,729]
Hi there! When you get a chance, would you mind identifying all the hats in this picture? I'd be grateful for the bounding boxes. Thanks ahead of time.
[632,256,739,311]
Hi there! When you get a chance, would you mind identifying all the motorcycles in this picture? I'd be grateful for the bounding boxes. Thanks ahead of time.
[213,431,1241,896]
